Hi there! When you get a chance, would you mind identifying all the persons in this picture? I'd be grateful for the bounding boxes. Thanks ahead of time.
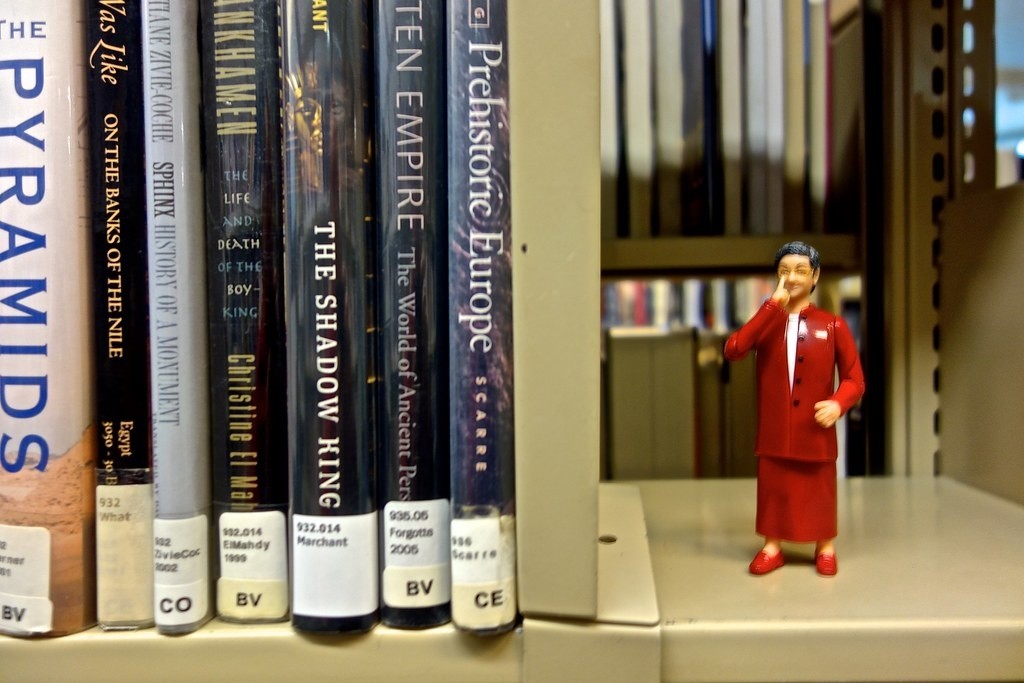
[722,242,864,577]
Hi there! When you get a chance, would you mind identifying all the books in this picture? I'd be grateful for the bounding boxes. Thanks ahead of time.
[602,275,882,482]
[598,0,838,245]
[0,1,522,646]
